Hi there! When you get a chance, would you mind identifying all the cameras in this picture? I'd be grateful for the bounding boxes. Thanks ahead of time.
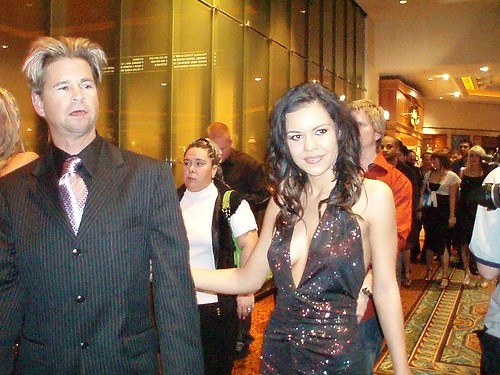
[467,184,500,210]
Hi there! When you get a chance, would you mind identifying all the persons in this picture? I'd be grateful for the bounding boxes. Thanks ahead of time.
[150,82,412,375]
[175,138,258,375]
[206,122,266,362]
[0,36,204,375]
[345,98,500,375]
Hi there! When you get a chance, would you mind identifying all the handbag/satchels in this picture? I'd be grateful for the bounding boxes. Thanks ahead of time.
[222,189,273,282]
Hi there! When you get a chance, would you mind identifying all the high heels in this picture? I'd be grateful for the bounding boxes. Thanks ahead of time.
[425,268,433,280]
[440,277,449,288]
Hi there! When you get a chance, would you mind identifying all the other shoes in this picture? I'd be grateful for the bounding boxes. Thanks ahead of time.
[405,275,411,287]
[477,278,487,288]
[463,271,471,286]
[235,340,249,359]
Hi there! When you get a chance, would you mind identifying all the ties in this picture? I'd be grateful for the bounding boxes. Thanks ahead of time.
[58,156,89,236]
